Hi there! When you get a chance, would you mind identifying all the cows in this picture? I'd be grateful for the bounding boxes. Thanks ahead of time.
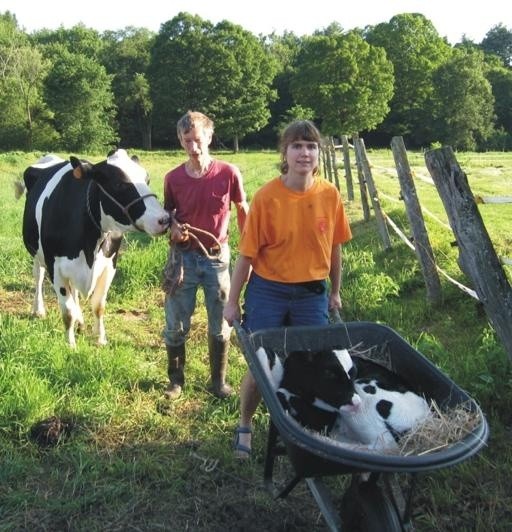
[255,345,441,457]
[14,148,171,350]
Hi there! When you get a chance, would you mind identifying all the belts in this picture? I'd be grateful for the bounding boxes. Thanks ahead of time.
[188,243,228,256]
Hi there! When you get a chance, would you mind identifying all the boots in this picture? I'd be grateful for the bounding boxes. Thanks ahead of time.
[207,333,236,400]
[165,343,186,401]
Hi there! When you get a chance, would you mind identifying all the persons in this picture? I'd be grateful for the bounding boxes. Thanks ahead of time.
[221,119,357,463]
[161,110,253,402]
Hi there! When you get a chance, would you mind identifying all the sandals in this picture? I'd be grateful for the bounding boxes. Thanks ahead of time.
[232,426,253,464]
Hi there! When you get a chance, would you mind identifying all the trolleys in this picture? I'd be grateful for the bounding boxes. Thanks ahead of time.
[232,307,489,532]
